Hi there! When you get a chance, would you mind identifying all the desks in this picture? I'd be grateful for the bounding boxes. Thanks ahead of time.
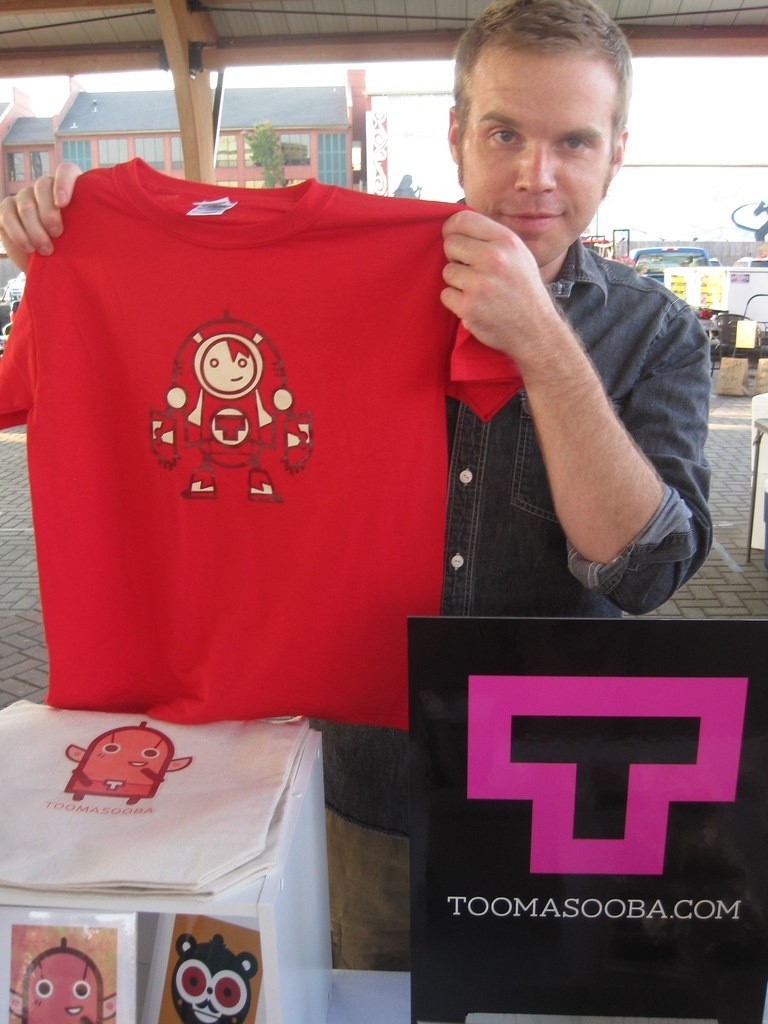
[698,315,718,377]
[746,419,768,562]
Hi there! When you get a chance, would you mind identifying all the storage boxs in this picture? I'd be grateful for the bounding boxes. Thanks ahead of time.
[0,730,333,1024]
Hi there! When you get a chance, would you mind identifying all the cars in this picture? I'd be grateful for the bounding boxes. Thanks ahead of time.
[629,247,768,284]
[10,271,27,300]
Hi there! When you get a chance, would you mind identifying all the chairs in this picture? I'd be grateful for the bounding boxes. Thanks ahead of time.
[751,393,768,550]
[715,313,762,368]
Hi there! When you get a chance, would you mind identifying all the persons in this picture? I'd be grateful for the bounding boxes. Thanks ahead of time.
[0,0,711,1023]
